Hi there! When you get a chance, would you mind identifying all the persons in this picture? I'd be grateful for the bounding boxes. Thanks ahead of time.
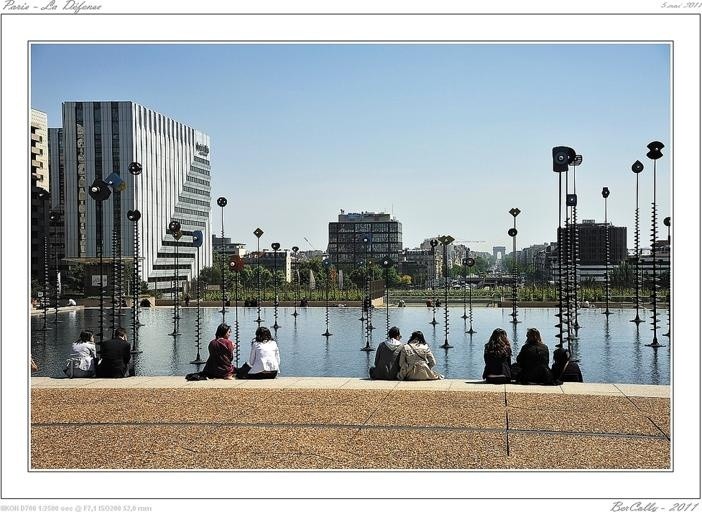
[426,297,441,307]
[552,349,583,382]
[245,296,257,306]
[203,324,236,378]
[581,299,590,308]
[300,298,309,307]
[370,326,404,380]
[64,329,99,377]
[482,329,512,384]
[247,326,280,379]
[66,297,77,306]
[516,328,558,384]
[395,331,439,380]
[96,328,132,377]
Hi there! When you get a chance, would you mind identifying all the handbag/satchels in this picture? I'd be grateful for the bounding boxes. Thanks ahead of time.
[407,342,427,361]
[557,361,570,379]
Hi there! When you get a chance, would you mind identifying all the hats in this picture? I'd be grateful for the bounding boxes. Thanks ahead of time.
[554,347,568,353]
